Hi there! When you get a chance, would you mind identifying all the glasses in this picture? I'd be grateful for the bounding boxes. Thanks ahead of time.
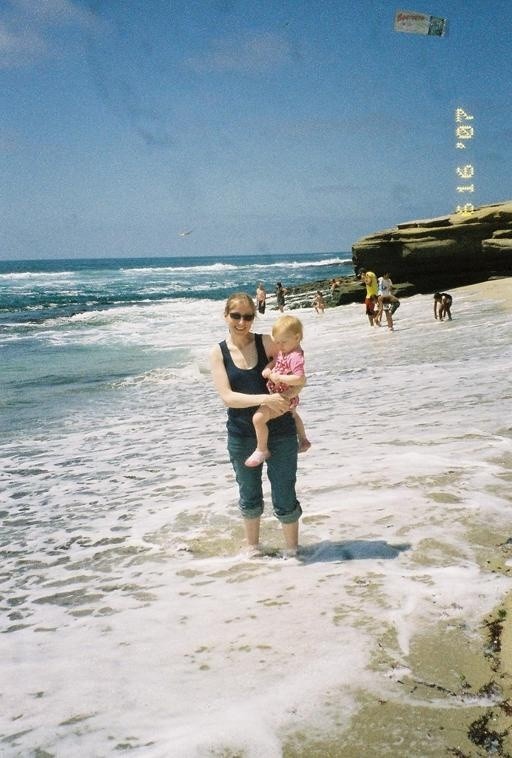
[230,312,253,320]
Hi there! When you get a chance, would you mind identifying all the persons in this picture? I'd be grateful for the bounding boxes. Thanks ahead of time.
[244,315,312,467]
[211,293,303,557]
[256,266,452,330]
